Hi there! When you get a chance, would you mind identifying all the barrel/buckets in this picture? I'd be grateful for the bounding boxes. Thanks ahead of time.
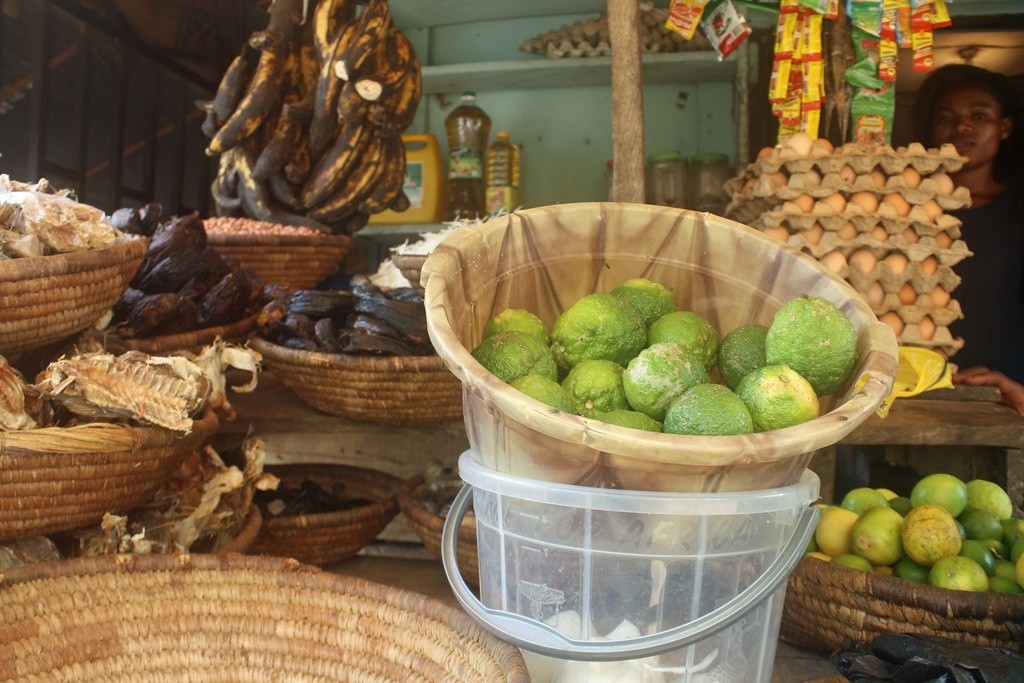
[438,447,823,682]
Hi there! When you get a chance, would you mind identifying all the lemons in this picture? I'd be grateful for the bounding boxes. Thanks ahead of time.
[467,276,858,440]
[802,473,1024,594]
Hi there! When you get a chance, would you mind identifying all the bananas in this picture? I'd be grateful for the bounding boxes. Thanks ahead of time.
[192,0,422,235]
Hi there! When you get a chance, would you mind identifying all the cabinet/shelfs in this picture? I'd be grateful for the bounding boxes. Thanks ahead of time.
[345,0,759,283]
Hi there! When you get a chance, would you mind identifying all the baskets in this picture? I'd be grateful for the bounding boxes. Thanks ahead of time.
[0,234,482,586]
[746,546,1024,654]
[0,553,531,683]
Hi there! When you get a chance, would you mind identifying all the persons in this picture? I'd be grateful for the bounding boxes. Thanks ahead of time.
[871,65,1024,498]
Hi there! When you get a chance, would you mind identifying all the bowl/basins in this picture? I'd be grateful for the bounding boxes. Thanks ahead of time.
[419,201,900,492]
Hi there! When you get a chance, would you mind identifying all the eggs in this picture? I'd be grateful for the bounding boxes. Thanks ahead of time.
[740,133,955,340]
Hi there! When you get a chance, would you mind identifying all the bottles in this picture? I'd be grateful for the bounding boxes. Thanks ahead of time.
[441,90,492,222]
[486,131,521,217]
[368,133,440,224]
